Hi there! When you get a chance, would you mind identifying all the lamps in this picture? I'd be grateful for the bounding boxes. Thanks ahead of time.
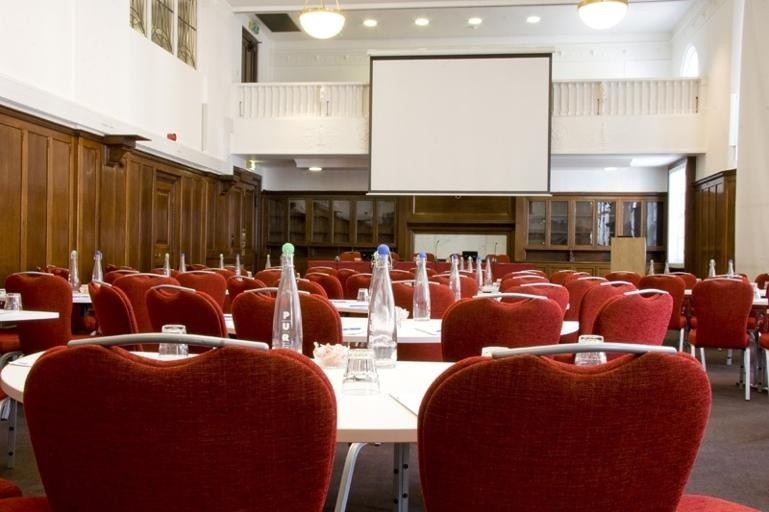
[576,0,632,34]
[298,2,348,40]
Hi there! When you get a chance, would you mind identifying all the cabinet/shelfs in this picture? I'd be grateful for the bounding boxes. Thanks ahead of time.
[514,192,667,277]
[256,190,400,278]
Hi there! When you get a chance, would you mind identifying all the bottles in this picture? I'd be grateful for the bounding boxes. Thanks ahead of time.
[219,253,224,268]
[67,250,80,288]
[663,261,670,275]
[91,250,103,282]
[412,250,432,320]
[163,253,172,276]
[265,254,271,268]
[467,256,473,274]
[178,252,187,273]
[708,259,717,276]
[272,254,303,356]
[235,254,242,276]
[484,256,492,287]
[366,243,397,369]
[475,256,483,288]
[449,254,461,301]
[459,256,464,270]
[727,259,734,274]
[648,259,654,275]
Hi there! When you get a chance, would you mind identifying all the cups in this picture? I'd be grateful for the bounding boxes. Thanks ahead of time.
[343,348,380,394]
[574,334,607,366]
[356,288,368,302]
[3,292,23,314]
[0,289,6,298]
[157,324,189,360]
